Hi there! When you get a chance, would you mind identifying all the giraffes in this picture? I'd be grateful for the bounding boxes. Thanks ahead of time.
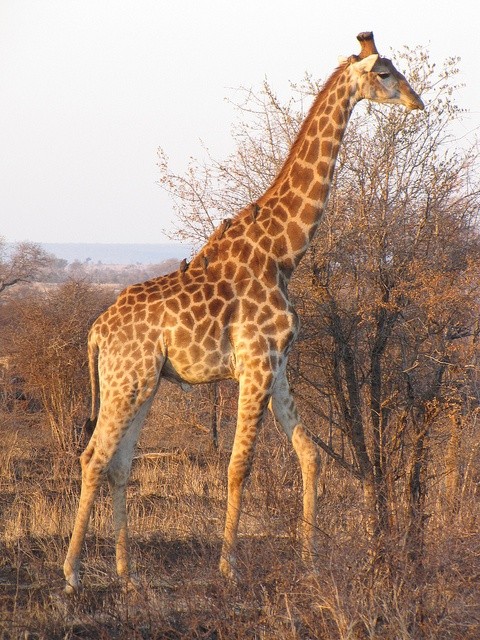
[61,31,425,595]
